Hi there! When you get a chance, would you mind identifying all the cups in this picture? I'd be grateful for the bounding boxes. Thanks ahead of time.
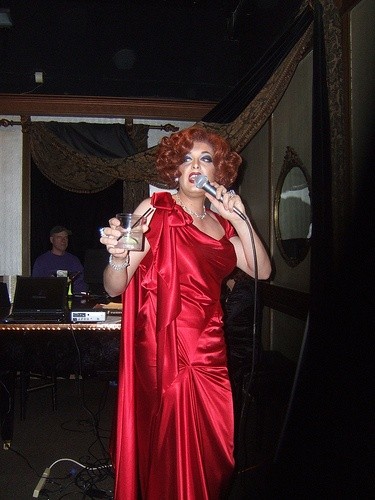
[117,212,145,251]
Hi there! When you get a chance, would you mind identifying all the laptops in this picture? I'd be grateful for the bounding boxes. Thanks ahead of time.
[1,277,67,324]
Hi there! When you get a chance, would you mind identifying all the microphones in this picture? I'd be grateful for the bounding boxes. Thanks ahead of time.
[194,175,246,220]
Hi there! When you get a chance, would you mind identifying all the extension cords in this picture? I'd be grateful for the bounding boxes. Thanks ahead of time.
[32,467,50,497]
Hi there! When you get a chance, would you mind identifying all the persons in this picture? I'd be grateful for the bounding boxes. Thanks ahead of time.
[32,225,89,298]
[100,125,272,500]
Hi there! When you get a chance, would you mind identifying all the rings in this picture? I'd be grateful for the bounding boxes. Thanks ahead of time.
[222,187,227,195]
[227,189,235,198]
[100,226,108,238]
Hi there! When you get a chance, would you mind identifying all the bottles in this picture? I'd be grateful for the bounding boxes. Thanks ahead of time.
[67,277,74,298]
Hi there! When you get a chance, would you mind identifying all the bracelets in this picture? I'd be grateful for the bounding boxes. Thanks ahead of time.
[108,254,130,269]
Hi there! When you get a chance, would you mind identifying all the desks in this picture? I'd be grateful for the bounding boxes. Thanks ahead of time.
[0,315,123,423]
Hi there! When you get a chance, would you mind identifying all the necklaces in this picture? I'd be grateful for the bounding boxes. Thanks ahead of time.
[174,193,207,220]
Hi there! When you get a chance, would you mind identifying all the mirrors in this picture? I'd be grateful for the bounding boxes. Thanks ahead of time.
[274,145,312,268]
